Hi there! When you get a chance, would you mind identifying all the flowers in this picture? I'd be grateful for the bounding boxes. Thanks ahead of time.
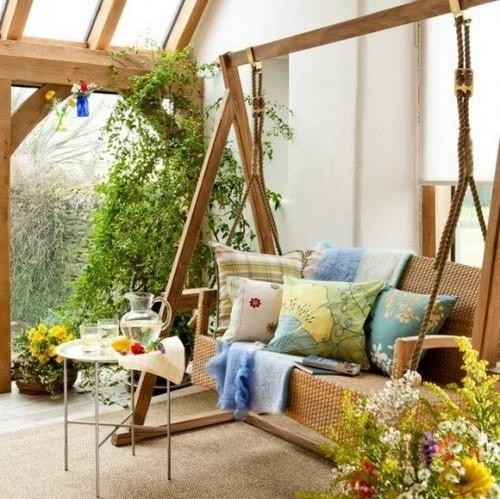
[111,335,166,358]
[12,323,77,401]
[43,89,76,134]
[291,368,500,499]
[72,78,104,97]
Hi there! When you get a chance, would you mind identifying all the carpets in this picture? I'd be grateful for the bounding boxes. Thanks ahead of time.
[1,387,344,499]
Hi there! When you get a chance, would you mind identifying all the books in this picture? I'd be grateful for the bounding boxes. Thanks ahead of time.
[294,361,345,376]
[302,354,363,376]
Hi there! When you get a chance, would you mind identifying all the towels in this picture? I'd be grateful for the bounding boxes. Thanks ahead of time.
[119,340,186,387]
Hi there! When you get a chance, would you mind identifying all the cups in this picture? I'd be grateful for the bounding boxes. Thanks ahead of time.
[79,323,100,353]
[97,318,119,353]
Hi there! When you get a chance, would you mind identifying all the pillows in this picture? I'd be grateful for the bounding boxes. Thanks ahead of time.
[207,240,463,381]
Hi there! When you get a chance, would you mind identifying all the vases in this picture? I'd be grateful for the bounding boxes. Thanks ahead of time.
[76,95,89,117]
[54,110,67,132]
[18,366,78,395]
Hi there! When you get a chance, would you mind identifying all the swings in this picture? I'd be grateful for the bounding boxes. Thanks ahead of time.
[190,12,500,464]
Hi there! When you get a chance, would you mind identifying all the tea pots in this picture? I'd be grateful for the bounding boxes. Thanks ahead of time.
[120,291,172,347]
